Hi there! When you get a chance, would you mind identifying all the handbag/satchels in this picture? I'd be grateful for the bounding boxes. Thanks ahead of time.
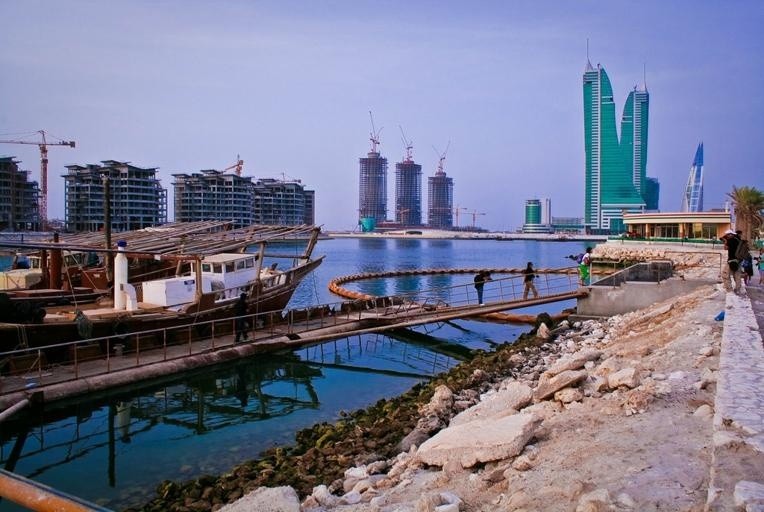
[728,261,739,271]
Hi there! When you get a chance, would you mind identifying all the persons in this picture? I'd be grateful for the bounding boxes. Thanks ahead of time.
[474,271,492,306]
[719,230,764,295]
[579,247,593,286]
[523,262,538,299]
[234,293,248,342]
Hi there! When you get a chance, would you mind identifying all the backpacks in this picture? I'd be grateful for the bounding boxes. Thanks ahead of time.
[735,237,750,260]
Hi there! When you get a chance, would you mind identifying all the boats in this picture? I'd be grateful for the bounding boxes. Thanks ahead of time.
[1,219,327,372]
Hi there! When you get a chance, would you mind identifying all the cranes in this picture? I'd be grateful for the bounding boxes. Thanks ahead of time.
[0,129,75,230]
[396,206,410,227]
[275,171,301,184]
[427,206,486,228]
[220,153,243,177]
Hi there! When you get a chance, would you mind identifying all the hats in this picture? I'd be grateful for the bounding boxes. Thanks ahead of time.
[725,230,737,235]
